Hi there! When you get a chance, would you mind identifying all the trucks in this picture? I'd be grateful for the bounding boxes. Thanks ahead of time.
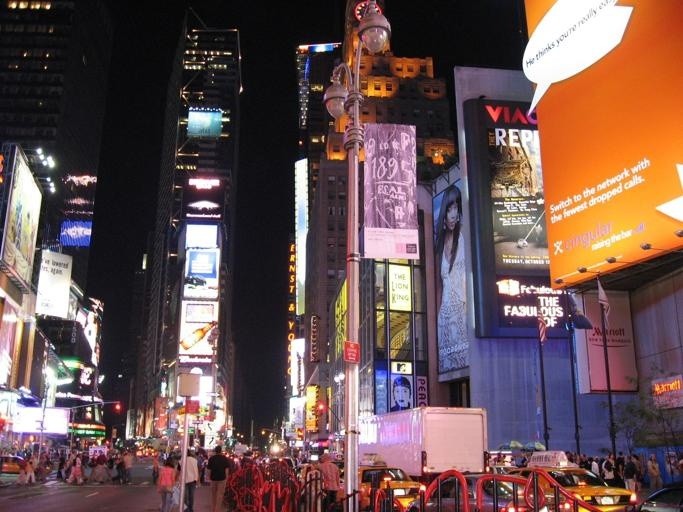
[358,407,487,477]
[88,446,107,466]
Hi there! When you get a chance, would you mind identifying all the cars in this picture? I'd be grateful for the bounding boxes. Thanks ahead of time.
[0,455,24,473]
[262,457,297,476]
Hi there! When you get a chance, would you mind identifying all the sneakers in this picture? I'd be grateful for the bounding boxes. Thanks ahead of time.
[15,475,135,486]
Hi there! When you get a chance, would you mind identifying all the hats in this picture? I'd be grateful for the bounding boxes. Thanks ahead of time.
[318,453,332,459]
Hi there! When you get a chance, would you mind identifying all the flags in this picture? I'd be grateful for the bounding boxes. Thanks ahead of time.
[567,291,593,329]
[597,277,611,331]
[537,299,548,345]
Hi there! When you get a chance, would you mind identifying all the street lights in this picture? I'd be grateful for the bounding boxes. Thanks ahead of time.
[322,1,391,512]
[261,426,284,440]
[65,401,121,450]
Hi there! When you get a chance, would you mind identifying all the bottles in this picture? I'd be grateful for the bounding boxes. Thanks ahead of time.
[179,320,218,351]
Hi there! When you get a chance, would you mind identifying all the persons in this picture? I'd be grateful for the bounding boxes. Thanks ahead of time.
[389,376,412,411]
[493,449,682,492]
[435,184,469,374]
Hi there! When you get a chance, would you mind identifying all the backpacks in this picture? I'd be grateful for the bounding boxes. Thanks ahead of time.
[604,460,613,472]
